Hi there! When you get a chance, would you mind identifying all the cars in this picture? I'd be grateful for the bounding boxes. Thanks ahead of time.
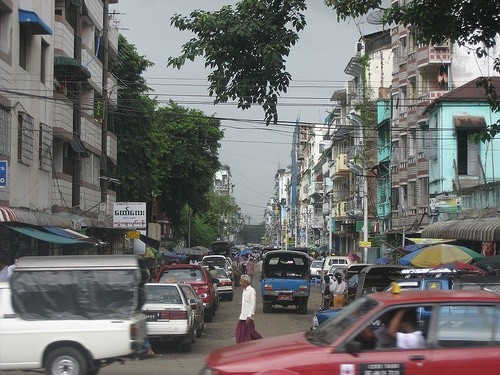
[140,239,250,354]
[198,281,500,375]
[310,255,417,306]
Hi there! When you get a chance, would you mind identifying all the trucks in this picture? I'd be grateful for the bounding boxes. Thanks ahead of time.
[258,250,313,314]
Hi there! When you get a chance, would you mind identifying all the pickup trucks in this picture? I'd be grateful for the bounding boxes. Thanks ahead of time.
[0,253,149,375]
[309,269,500,332]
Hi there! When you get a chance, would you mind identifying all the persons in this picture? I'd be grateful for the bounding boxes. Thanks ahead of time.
[235,274,263,344]
[359,311,396,350]
[232,247,260,289]
[319,273,349,311]
[0,254,22,281]
[308,251,327,262]
[386,308,427,349]
[136,282,154,356]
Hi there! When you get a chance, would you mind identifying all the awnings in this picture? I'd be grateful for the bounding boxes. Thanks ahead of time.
[54,56,91,79]
[421,217,500,240]
[19,10,53,36]
[0,207,111,245]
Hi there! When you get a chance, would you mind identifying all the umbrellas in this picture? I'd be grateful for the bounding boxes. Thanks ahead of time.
[376,257,392,266]
[137,246,159,260]
[398,237,487,284]
